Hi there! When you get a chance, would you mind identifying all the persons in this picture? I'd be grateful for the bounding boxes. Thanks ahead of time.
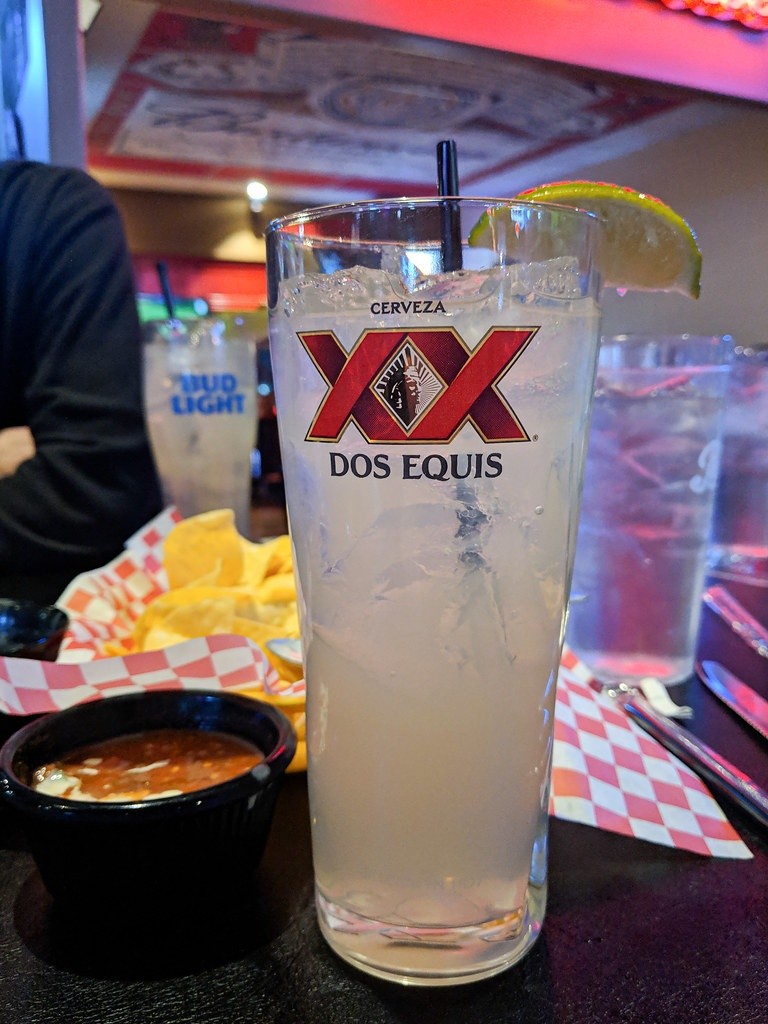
[0,150,167,658]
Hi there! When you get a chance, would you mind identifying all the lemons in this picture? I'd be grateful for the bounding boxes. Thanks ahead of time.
[467,179,701,300]
[215,311,270,339]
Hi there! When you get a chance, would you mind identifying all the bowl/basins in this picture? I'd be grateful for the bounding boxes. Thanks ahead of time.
[0,689,298,922]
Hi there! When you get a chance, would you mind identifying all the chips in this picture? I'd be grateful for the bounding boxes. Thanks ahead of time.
[104,507,300,683]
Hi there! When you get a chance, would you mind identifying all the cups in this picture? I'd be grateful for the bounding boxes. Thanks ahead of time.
[144,314,255,539]
[566,331,734,691]
[266,196,606,987]
[706,352,768,586]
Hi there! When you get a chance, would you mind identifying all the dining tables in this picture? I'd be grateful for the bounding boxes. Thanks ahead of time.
[0,583,766,1023]
